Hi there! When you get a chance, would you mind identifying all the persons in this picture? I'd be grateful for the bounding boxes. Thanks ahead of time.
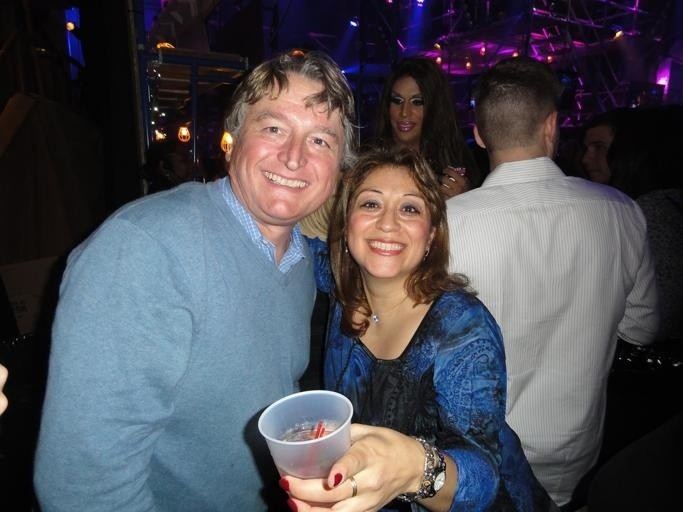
[296,55,683,512]
[279,145,539,512]
[30,48,365,511]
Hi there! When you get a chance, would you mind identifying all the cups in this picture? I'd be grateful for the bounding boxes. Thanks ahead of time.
[258,389,354,497]
